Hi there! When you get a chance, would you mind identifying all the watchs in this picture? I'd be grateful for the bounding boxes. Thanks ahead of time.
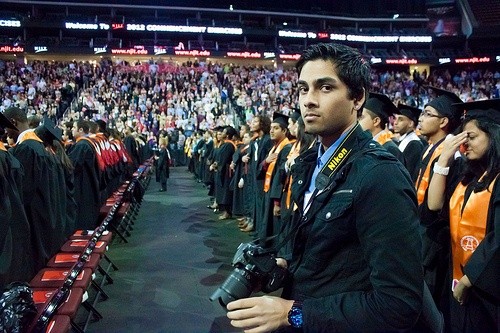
[288,299,304,329]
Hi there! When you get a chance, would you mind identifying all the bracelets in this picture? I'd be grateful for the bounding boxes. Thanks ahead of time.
[433,161,450,175]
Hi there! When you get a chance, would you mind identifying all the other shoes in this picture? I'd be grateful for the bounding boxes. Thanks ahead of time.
[207,205,253,232]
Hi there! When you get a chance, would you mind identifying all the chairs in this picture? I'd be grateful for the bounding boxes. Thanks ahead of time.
[28,153,155,333]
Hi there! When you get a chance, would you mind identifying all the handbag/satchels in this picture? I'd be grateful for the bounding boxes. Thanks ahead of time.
[0,286,44,333]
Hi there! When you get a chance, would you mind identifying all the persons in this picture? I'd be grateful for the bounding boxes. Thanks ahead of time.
[415,85,466,332]
[256,111,292,241]
[427,97,500,332]
[0,57,255,232]
[356,92,407,172]
[286,107,313,227]
[226,42,424,333]
[256,57,303,112]
[394,101,424,183]
[0,111,34,291]
[292,111,311,223]
[3,106,52,274]
[368,59,499,110]
[34,116,67,258]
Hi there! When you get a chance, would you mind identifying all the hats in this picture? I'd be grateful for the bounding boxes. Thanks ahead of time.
[363,91,403,122]
[0,112,19,135]
[427,85,464,115]
[34,115,62,142]
[289,107,301,119]
[451,98,500,122]
[397,103,421,120]
[272,112,289,127]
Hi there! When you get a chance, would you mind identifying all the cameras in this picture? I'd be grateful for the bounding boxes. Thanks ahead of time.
[210,242,290,309]
